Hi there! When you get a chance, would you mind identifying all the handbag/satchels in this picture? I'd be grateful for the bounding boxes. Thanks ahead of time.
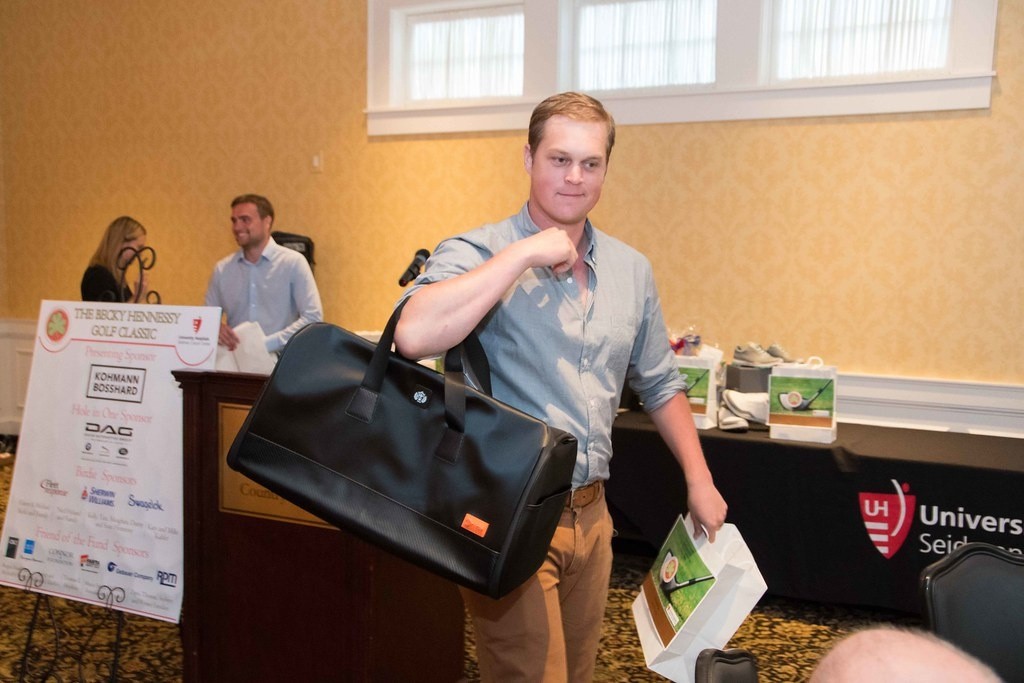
[227,287,582,605]
[672,353,719,430]
[629,505,768,683]
[763,360,837,444]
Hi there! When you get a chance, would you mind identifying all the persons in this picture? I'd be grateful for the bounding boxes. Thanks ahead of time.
[391,91,728,683]
[81,215,150,302]
[203,192,322,353]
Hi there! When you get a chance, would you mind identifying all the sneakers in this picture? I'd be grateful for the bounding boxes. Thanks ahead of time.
[731,341,783,369]
[764,343,804,365]
[721,387,770,425]
[714,405,748,433]
